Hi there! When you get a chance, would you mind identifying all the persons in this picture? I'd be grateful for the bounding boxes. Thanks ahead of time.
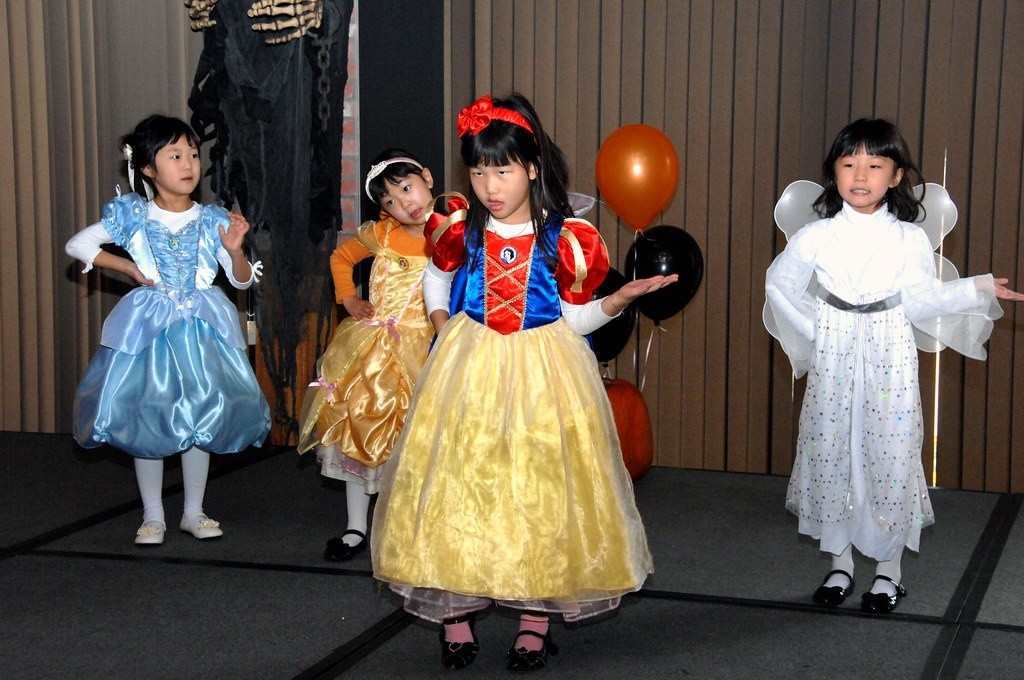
[296,150,435,562]
[761,118,1023,611]
[63,113,272,545]
[369,92,677,673]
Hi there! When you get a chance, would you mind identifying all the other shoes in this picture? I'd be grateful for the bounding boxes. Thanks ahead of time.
[134,518,167,544]
[439,615,480,668]
[506,630,549,673]
[181,513,223,539]
[323,529,368,562]
[812,570,856,607]
[862,575,908,613]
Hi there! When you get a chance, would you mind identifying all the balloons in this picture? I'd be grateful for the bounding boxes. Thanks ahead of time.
[624,225,705,328]
[590,266,636,367]
[594,124,679,231]
